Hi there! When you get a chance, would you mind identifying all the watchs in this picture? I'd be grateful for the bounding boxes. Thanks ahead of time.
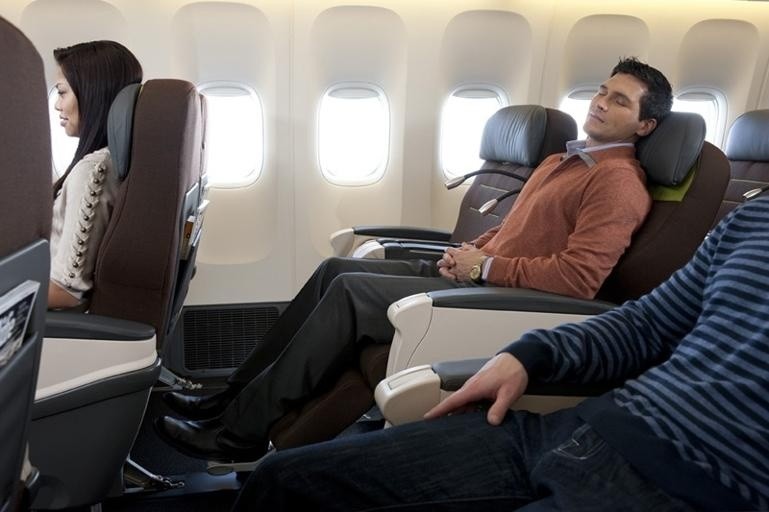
[469,253,489,284]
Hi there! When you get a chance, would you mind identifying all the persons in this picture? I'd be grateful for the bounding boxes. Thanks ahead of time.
[232,183,769,512]
[156,58,674,462]
[47,40,142,314]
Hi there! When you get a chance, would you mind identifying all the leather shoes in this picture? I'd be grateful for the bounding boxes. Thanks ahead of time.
[151,413,270,464]
[161,383,238,423]
